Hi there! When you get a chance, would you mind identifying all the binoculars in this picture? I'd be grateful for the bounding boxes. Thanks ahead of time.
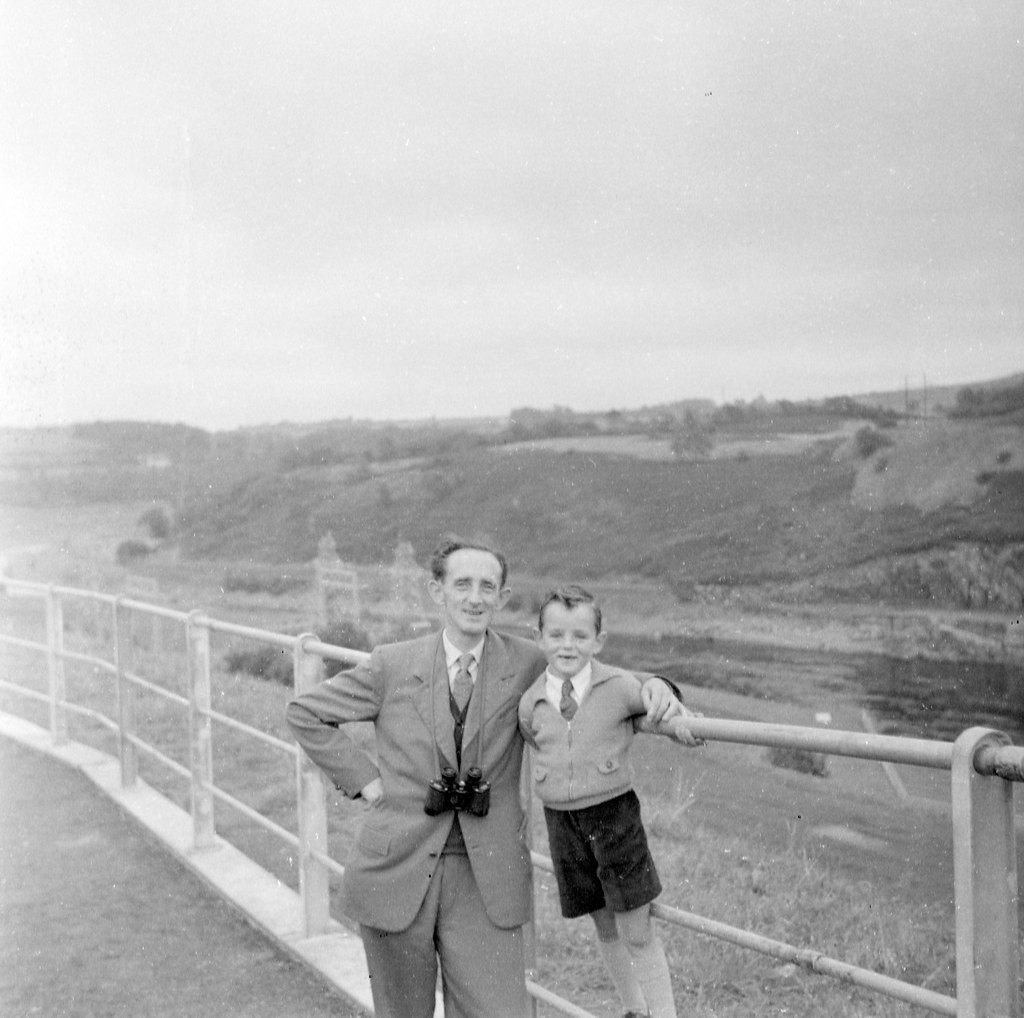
[422,767,492,818]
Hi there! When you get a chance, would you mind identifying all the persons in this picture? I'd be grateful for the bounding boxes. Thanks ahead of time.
[285,539,688,1017]
[517,584,705,1018]
